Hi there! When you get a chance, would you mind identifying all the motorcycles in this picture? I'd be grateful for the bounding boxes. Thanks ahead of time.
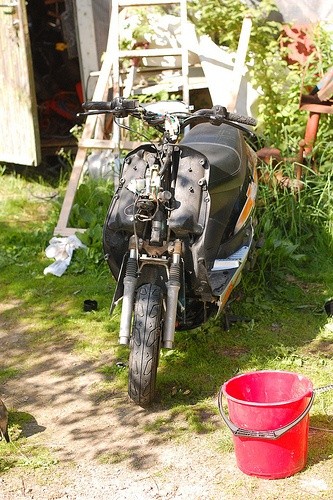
[76,96,259,407]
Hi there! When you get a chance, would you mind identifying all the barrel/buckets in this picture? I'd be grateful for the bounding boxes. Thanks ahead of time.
[218,370,315,480]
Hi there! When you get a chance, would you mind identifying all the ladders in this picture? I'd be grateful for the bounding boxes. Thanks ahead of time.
[53,0,192,249]
